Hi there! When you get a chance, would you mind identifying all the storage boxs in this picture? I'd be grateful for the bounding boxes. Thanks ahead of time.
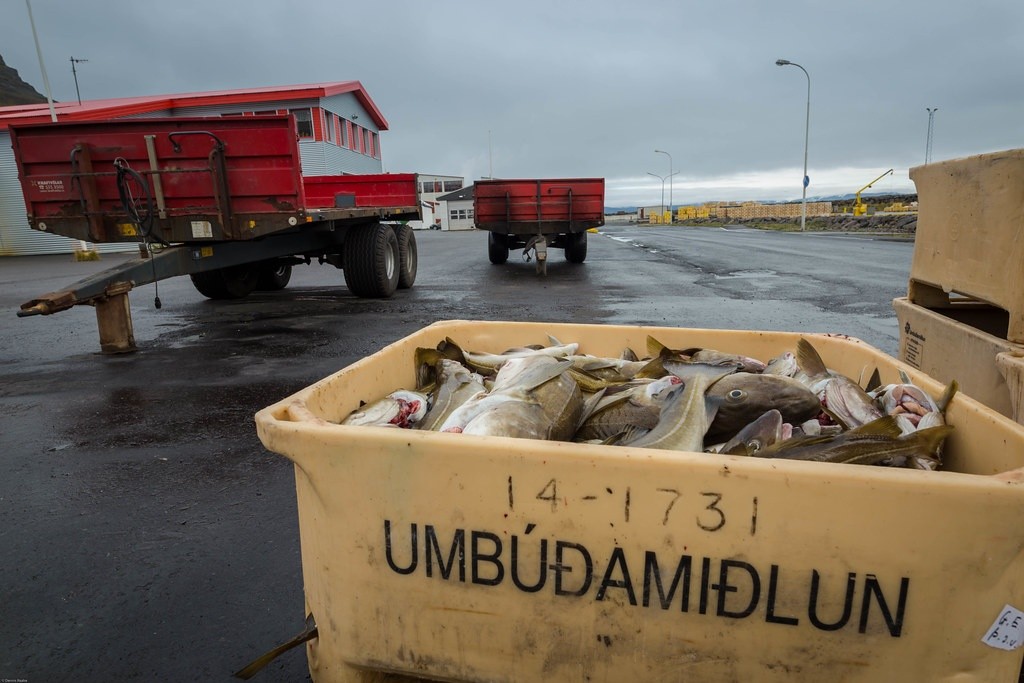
[892,296,1024,427]
[905,149,1024,346]
[259,318,1024,683]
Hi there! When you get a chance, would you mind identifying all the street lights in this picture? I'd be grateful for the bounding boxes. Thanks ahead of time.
[775,59,811,235]
[647,172,679,224]
[655,149,673,224]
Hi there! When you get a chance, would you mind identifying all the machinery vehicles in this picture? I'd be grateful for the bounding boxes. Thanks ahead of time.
[853,168,894,217]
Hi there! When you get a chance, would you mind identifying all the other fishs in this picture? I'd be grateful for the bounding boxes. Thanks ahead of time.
[285,332,958,469]
